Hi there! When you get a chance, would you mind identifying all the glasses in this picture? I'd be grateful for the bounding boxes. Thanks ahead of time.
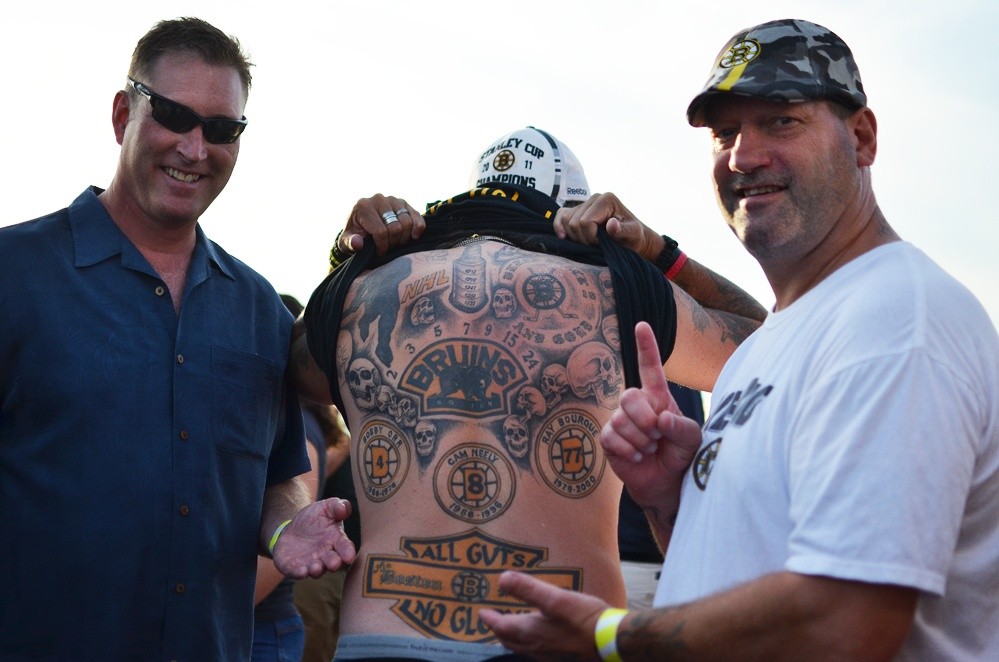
[126,74,248,144]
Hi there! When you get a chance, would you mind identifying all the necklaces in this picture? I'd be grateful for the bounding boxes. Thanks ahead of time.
[452,236,515,248]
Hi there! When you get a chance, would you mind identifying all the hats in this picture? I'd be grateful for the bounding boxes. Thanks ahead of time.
[687,18,868,128]
[473,125,592,208]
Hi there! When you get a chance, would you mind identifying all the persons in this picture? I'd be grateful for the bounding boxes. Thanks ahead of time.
[478,19,999,662]
[0,19,359,662]
[254,127,770,662]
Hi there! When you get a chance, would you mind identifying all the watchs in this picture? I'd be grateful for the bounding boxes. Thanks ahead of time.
[655,234,678,269]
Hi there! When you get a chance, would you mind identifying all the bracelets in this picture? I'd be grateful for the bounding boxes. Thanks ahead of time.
[664,252,688,280]
[329,229,351,269]
[269,519,293,555]
[661,247,681,273]
[595,607,629,662]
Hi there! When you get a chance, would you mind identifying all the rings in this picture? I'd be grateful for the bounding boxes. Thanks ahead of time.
[395,208,408,216]
[381,210,398,225]
